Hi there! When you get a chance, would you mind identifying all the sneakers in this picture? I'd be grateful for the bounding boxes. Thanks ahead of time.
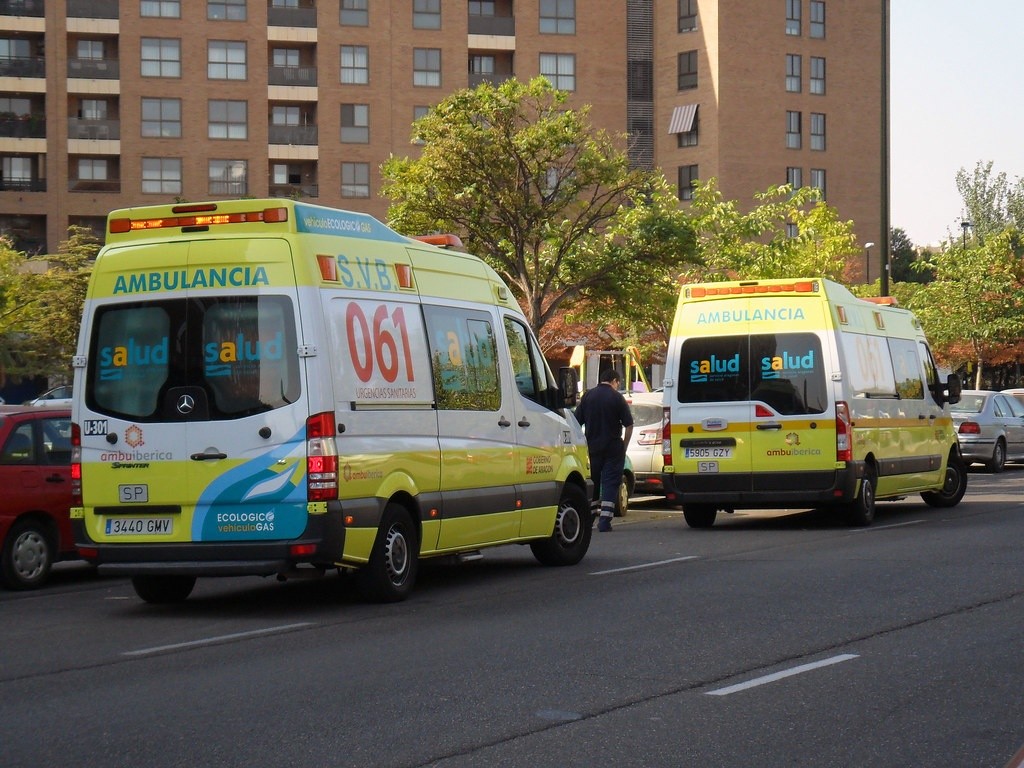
[599,526,613,532]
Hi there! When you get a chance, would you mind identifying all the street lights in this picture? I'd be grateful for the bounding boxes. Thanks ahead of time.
[960,220,974,252]
[863,242,873,288]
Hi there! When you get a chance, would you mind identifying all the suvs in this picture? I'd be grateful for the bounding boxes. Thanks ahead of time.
[614,393,666,492]
[0,404,90,588]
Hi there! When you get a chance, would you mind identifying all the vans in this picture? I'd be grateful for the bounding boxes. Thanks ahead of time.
[66,197,599,614]
[661,275,970,526]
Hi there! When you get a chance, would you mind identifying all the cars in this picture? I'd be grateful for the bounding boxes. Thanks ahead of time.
[1003,388,1024,404]
[948,388,1023,473]
[25,383,73,405]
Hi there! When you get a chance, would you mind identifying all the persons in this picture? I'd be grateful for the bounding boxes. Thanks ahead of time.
[574,369,634,532]
